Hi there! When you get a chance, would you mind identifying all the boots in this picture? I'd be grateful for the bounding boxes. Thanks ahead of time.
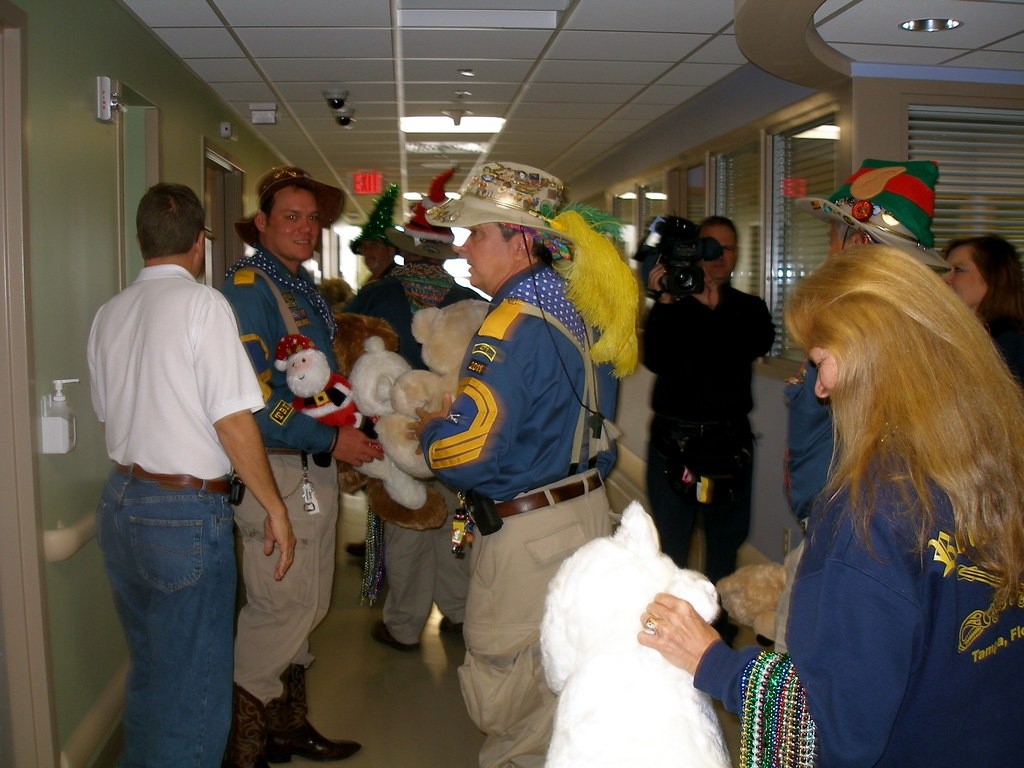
[221,683,270,768]
[265,665,362,762]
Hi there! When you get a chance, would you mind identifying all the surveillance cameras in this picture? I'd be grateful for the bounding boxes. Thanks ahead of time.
[323,87,349,109]
[333,107,355,125]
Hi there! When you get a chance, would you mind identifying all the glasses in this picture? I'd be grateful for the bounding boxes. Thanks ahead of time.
[195,227,216,246]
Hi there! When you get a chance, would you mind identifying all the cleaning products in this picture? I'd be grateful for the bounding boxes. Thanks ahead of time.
[47,378,79,453]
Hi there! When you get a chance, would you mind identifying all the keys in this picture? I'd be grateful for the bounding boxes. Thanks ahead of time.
[304,482,313,501]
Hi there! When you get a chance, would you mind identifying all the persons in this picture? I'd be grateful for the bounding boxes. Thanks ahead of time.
[783,160,939,529]
[218,167,385,768]
[403,163,636,767]
[639,216,775,647]
[941,235,1024,390]
[321,166,474,653]
[637,245,1024,768]
[87,183,297,767]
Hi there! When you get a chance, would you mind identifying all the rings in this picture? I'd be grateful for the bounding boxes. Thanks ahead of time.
[411,432,417,440]
[646,618,658,629]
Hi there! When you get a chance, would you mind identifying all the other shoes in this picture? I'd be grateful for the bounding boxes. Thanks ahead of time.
[440,616,464,634]
[373,620,419,651]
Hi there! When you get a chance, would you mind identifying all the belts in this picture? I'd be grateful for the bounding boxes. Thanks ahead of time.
[116,464,229,493]
[654,414,745,436]
[493,473,601,518]
[263,448,311,456]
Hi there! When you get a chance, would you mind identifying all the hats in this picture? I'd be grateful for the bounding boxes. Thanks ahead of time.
[235,166,344,248]
[796,159,951,271]
[349,186,400,255]
[386,168,462,260]
[425,162,639,379]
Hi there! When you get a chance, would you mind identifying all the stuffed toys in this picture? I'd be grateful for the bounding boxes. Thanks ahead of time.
[274,333,379,468]
[376,300,492,479]
[351,337,427,509]
[540,501,731,768]
